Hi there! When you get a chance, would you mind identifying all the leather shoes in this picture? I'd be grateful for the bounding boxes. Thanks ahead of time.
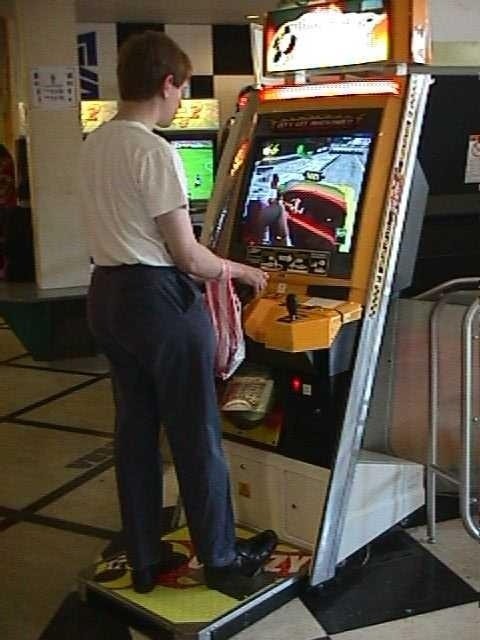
[131,554,191,592]
[214,527,280,580]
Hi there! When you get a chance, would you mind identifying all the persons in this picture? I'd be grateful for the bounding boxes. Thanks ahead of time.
[71,29,277,596]
[268,172,301,247]
[194,174,202,190]
[0,143,18,281]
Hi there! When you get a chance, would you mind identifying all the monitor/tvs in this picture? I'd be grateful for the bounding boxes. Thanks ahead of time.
[235,128,377,256]
[167,133,216,204]
